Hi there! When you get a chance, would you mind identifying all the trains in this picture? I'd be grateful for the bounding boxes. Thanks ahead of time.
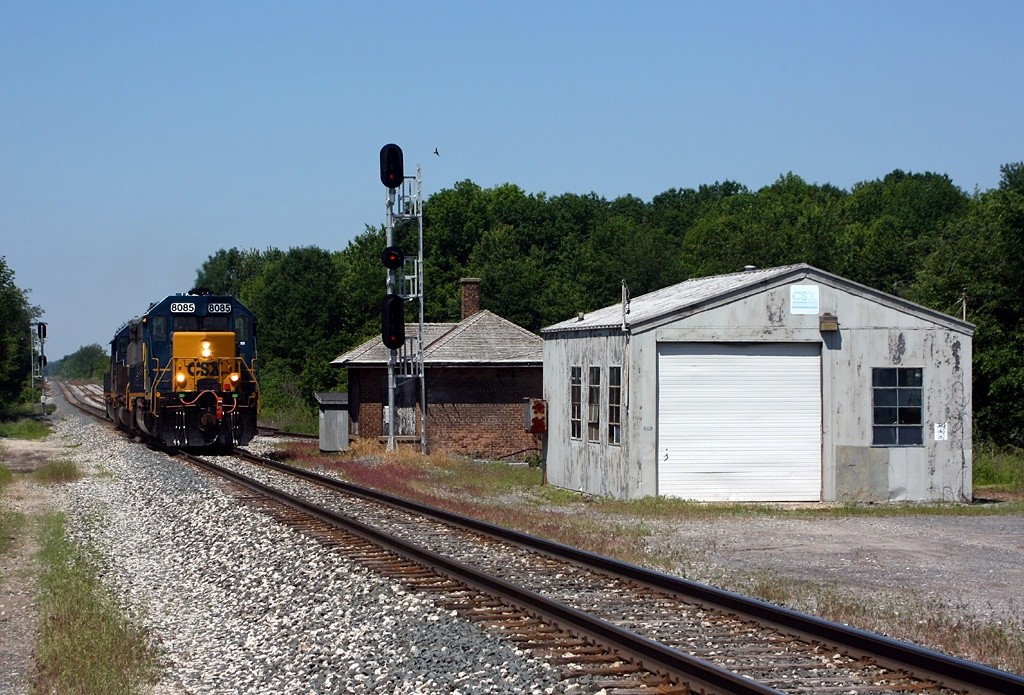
[103,288,260,451]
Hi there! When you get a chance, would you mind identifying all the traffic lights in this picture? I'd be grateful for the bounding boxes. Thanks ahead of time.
[381,295,405,350]
[381,246,404,270]
[380,143,404,188]
